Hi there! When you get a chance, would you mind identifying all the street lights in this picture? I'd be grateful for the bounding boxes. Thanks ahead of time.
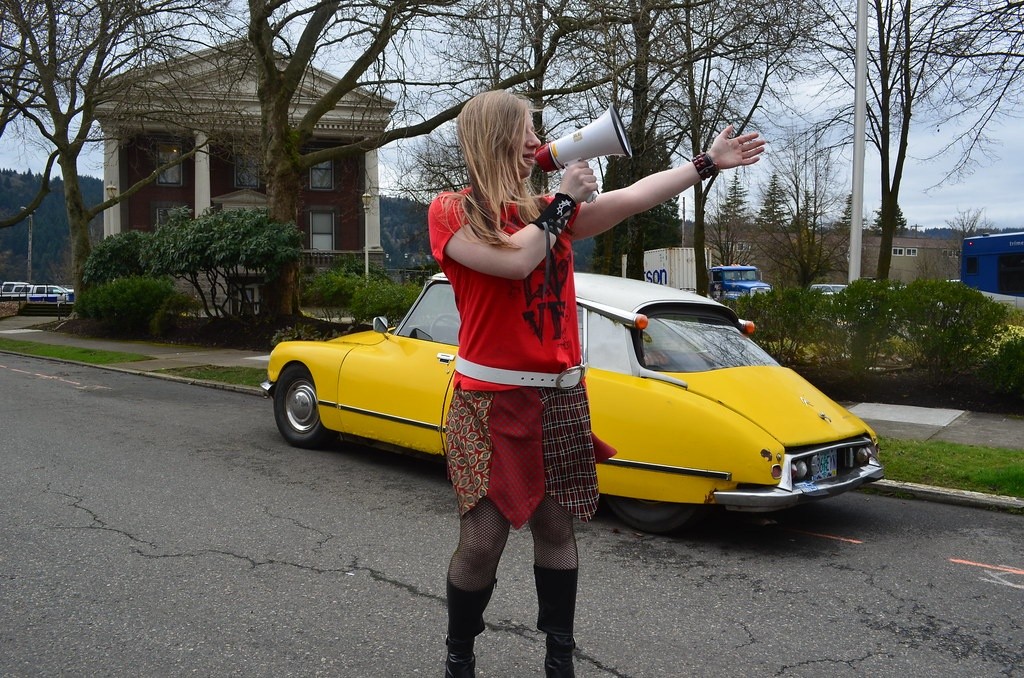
[362,192,372,275]
[385,253,431,283]
[20,206,36,282]
[106,180,117,234]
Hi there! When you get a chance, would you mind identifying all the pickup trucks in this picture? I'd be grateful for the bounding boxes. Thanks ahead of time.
[0,282,74,302]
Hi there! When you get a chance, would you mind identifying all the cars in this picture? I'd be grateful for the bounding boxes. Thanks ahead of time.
[809,284,848,295]
[260,272,885,533]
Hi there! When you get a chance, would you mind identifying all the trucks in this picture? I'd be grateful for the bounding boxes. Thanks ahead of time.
[962,231,1024,311]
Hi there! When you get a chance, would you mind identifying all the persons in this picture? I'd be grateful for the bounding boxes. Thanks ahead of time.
[428,91,766,678]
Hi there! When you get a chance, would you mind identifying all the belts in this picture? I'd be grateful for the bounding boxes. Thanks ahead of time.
[453,355,588,390]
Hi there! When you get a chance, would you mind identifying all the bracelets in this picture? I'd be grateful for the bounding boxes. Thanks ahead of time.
[692,152,716,181]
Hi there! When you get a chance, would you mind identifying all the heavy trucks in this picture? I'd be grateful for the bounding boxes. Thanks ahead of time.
[643,245,773,302]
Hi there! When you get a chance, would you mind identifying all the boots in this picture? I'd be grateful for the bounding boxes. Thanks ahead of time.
[544,634,575,678]
[445,635,475,678]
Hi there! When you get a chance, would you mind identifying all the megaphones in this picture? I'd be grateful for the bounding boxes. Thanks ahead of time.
[535,104,632,203]
